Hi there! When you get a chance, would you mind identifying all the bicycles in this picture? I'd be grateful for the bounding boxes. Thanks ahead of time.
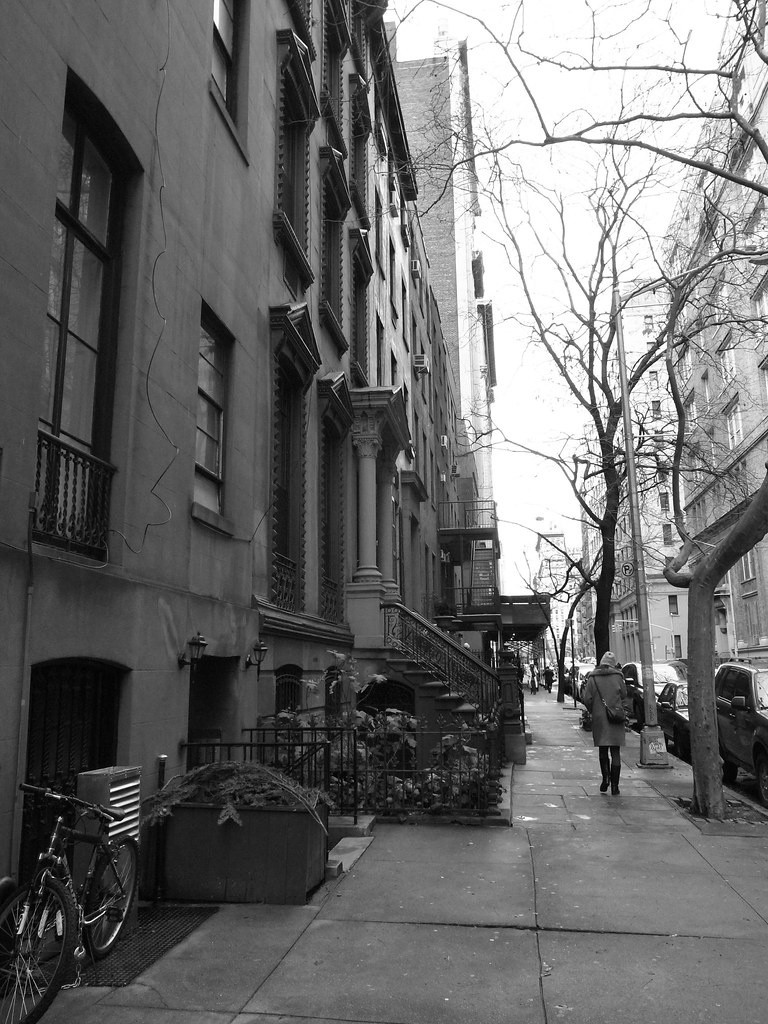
[0,783,140,1024]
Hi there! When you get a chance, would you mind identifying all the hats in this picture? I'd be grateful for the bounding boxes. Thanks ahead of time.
[600,652,616,669]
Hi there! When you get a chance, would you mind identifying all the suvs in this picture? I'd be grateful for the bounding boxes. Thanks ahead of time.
[714,657,768,808]
[620,661,687,731]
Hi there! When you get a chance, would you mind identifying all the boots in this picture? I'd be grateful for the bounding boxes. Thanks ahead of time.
[600,757,610,791]
[611,764,620,795]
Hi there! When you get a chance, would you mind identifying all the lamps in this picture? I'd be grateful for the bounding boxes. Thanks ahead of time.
[176,631,208,671]
[244,638,268,681]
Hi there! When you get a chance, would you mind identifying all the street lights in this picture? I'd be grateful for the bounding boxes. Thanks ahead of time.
[613,577,654,665]
[612,255,768,768]
[671,538,738,662]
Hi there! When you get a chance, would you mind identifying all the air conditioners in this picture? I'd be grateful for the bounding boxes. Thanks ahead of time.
[412,259,420,278]
[440,473,446,482]
[451,464,460,475]
[440,434,449,450]
[413,353,429,372]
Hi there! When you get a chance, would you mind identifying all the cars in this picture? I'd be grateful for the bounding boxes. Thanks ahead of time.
[554,657,597,702]
[656,681,691,760]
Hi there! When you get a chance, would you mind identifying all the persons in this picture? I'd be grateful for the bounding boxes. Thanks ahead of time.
[542,666,554,693]
[527,664,541,695]
[584,651,628,795]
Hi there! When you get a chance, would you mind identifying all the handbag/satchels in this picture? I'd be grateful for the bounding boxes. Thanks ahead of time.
[606,705,625,723]
